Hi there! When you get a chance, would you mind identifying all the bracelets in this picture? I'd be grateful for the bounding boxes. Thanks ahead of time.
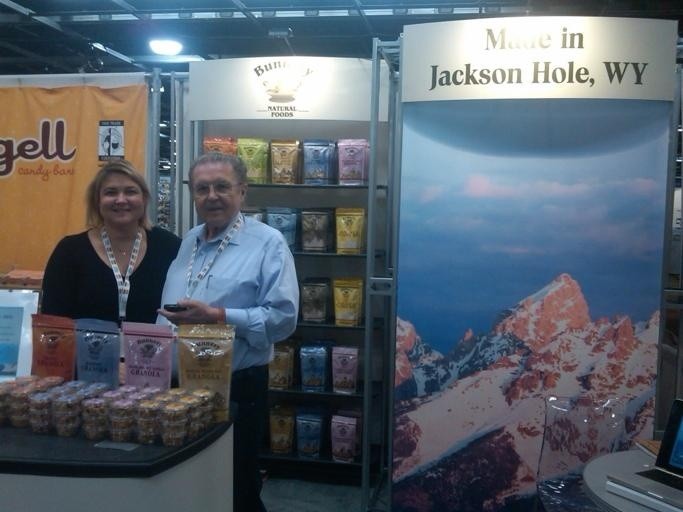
[218,307,225,321]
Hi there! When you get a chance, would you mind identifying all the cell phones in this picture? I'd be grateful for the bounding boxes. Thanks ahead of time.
[163,304,186,314]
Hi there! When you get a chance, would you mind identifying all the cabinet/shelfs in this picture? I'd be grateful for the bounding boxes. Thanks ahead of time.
[187,120,386,469]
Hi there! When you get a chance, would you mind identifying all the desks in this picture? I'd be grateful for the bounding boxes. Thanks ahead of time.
[581,447,683,512]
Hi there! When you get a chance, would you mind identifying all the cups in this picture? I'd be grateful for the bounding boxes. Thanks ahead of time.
[536,395,614,511]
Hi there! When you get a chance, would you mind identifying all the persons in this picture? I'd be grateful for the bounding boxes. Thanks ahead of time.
[41,159,182,362]
[154,151,300,512]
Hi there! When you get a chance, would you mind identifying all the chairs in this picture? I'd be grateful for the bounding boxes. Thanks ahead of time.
[535,394,626,512]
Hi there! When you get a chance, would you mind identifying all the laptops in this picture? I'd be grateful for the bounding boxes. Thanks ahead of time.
[607,398,683,512]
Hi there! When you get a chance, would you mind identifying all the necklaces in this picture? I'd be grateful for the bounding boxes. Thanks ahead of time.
[109,237,132,257]
